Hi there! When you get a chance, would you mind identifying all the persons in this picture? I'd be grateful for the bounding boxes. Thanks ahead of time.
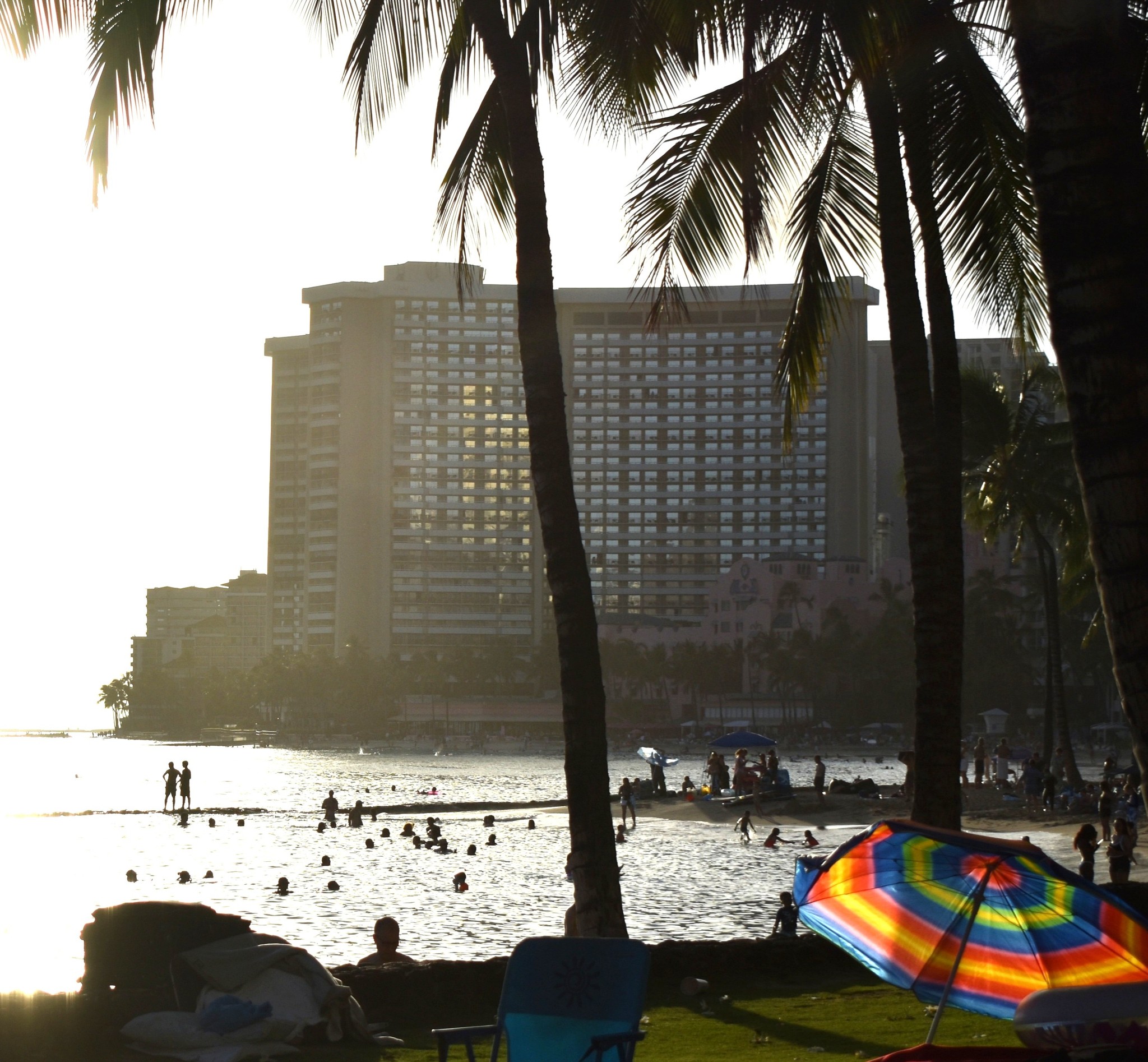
[162,761,182,811]
[322,790,338,819]
[1106,818,1137,902]
[275,822,375,896]
[616,825,627,842]
[735,811,757,840]
[178,761,191,810]
[649,735,1147,840]
[618,777,636,825]
[348,800,364,827]
[177,871,192,883]
[379,816,537,855]
[357,916,413,962]
[764,828,796,847]
[454,872,469,891]
[177,811,245,827]
[773,891,798,938]
[1074,824,1100,881]
[802,830,819,846]
[126,869,138,883]
[366,785,440,796]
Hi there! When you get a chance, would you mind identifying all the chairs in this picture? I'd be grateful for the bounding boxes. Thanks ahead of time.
[425,928,654,1062]
[169,958,211,1012]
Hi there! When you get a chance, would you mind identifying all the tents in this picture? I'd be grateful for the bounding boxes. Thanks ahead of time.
[700,731,777,789]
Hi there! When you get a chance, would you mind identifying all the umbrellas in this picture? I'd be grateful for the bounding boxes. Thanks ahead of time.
[793,818,1148,1044]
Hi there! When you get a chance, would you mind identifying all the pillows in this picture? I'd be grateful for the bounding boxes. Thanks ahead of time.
[200,993,274,1038]
[119,1009,221,1048]
